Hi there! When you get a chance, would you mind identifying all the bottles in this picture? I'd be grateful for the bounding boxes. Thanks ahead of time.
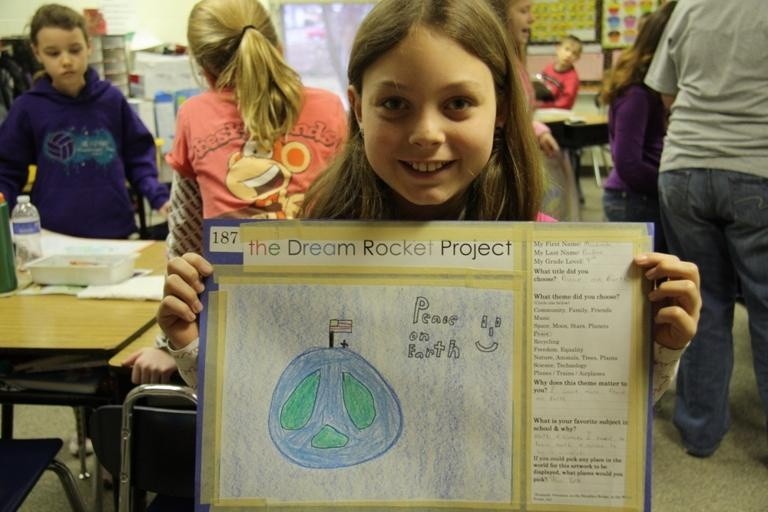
[11,195,42,271]
[0,192,18,296]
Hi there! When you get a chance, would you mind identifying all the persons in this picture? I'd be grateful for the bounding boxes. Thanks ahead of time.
[534,35,582,109]
[0,4,172,457]
[155,0,702,410]
[602,0,679,287]
[120,0,350,384]
[644,0,768,458]
[491,0,559,160]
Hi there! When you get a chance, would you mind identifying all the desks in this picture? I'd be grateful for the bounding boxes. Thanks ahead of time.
[545,109,609,186]
[109,314,179,375]
[1,240,168,512]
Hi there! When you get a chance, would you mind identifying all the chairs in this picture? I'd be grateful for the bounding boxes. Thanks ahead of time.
[562,123,608,206]
[88,383,198,511]
[0,438,90,512]
